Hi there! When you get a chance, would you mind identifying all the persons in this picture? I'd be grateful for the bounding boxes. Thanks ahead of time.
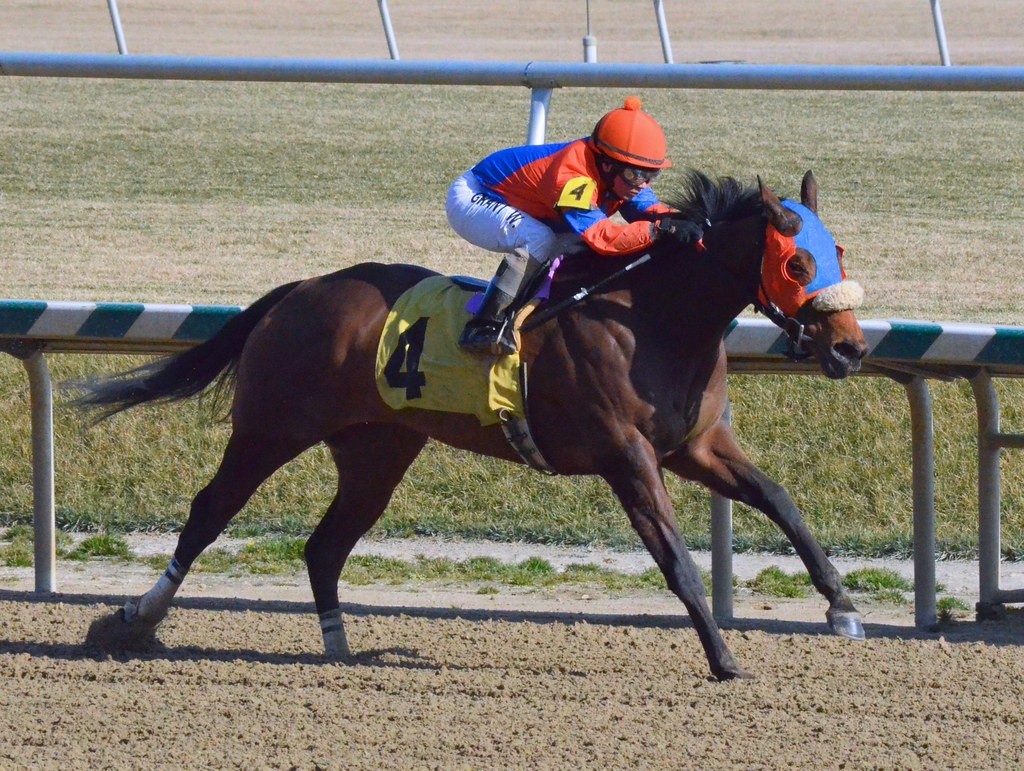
[446,96,708,352]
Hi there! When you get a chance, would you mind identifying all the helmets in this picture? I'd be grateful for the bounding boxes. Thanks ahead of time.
[588,95,673,169]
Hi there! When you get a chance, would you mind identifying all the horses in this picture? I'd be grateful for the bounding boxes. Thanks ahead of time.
[53,168,872,683]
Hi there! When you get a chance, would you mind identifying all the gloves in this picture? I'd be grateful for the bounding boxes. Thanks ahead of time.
[660,217,704,247]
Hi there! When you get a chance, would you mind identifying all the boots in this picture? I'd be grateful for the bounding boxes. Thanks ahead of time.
[457,248,542,355]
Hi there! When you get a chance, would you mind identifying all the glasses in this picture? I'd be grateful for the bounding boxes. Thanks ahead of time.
[594,154,662,187]
